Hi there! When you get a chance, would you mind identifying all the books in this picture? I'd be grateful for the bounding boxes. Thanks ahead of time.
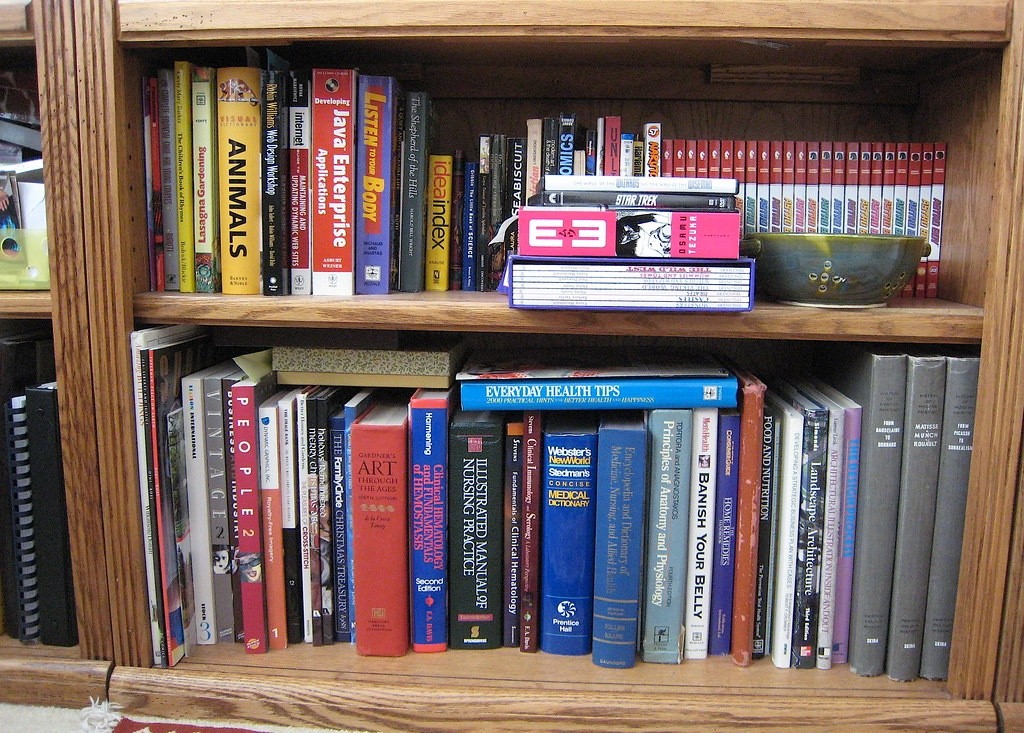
[140,60,949,312]
[129,322,981,682]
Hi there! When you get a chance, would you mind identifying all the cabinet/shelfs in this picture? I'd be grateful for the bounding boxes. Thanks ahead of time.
[1,1,1024,733]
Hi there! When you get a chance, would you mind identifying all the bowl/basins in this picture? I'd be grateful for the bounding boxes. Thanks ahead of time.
[738,233,932,308]
